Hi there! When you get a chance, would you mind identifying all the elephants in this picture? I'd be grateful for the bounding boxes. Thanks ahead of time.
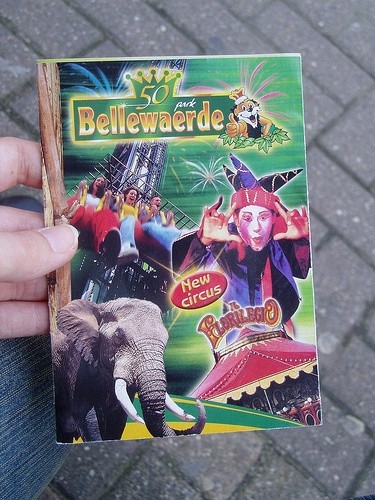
[50,298,206,444]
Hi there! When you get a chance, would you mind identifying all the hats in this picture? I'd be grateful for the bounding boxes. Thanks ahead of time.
[222,157,303,214]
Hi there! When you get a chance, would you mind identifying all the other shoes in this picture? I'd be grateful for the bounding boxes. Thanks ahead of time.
[116,246,139,270]
[103,225,122,268]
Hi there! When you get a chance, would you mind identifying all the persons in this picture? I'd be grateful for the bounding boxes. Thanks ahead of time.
[69,175,122,266]
[1,137,80,340]
[112,187,183,266]
[175,155,313,339]
